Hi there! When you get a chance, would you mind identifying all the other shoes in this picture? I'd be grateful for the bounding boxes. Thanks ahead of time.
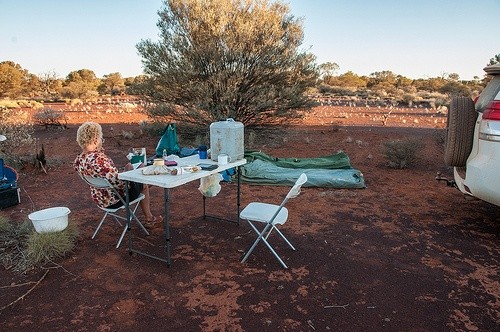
[145,214,165,228]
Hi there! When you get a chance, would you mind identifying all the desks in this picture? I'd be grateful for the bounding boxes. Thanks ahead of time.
[117,154,248,270]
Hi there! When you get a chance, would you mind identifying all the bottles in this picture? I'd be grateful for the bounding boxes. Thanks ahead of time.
[198,145,208,158]
[217,153,232,167]
[163,149,168,160]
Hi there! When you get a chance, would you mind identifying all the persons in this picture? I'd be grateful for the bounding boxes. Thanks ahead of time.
[73,122,163,225]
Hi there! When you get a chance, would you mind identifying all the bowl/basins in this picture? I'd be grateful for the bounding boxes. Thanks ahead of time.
[28,206,71,233]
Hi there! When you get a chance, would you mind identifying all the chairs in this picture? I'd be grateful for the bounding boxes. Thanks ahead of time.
[78,172,149,249]
[238,173,308,270]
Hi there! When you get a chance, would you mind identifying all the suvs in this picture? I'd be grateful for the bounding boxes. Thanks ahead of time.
[434,63,500,208]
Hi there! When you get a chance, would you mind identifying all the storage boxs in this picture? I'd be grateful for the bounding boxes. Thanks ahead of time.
[209,118,244,163]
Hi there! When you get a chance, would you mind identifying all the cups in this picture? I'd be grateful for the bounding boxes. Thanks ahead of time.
[153,159,164,165]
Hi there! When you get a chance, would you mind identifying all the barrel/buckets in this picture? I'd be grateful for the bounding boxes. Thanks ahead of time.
[209,118,245,162]
